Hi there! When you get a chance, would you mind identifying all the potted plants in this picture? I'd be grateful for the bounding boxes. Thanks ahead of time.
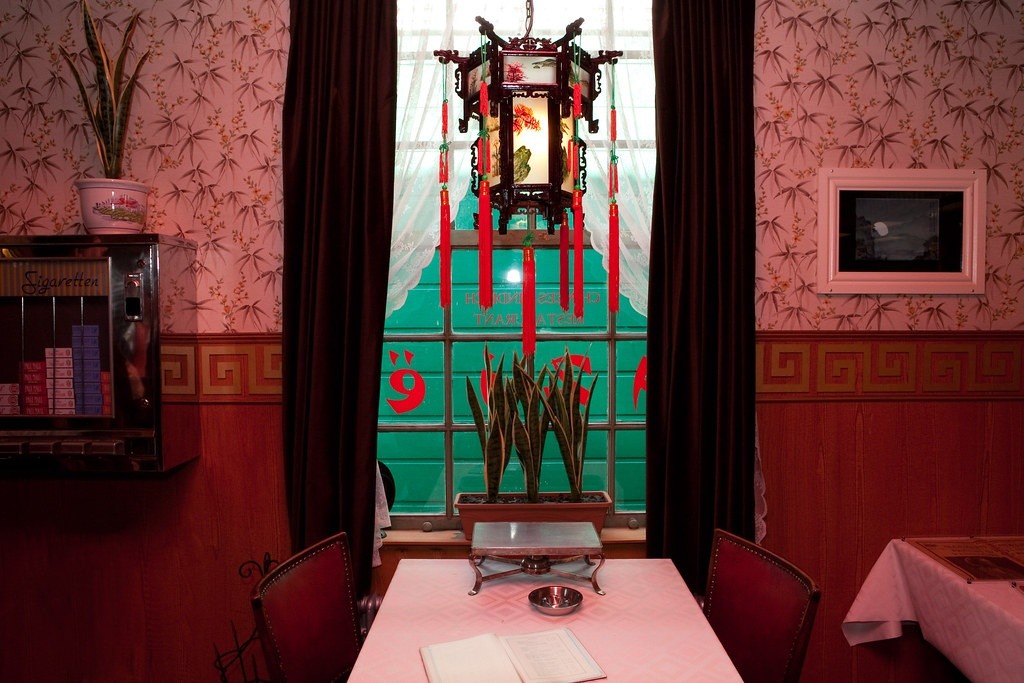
[62,0,151,235]
[454,339,613,539]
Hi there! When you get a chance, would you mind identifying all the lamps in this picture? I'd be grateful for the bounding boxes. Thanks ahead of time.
[430,0,625,359]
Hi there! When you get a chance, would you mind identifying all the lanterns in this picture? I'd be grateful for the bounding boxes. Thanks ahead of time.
[433,0,624,358]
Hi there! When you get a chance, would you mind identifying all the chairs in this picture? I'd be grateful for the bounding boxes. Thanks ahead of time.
[250,531,363,683]
[704,528,822,683]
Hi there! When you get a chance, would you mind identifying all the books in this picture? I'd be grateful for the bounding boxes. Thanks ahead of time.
[419,628,607,683]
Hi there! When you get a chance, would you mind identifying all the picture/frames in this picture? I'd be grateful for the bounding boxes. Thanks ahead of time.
[816,166,988,295]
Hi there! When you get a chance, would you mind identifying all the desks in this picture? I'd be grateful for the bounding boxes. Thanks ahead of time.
[843,537,1024,683]
[344,557,749,683]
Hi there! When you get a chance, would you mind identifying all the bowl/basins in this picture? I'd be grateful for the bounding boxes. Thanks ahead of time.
[528,586,583,615]
